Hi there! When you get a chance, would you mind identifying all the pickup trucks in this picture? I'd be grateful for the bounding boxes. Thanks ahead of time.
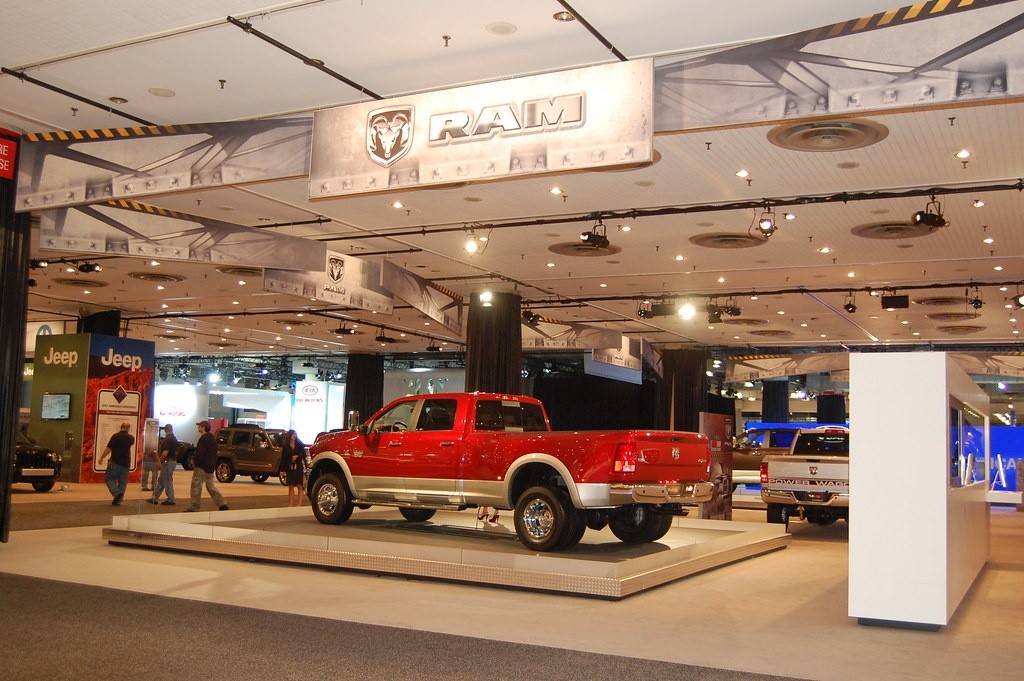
[306,393,715,551]
[760,426,849,534]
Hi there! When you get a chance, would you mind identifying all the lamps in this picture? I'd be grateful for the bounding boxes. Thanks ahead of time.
[426,338,442,351]
[464,228,493,256]
[78,262,103,273]
[725,296,741,317]
[706,298,723,324]
[969,286,985,309]
[1010,280,1024,310]
[480,283,493,307]
[301,357,314,368]
[335,319,354,334]
[522,308,542,325]
[580,219,609,250]
[637,299,653,318]
[376,327,395,343]
[748,208,778,242]
[29,259,48,270]
[844,291,857,314]
[916,195,950,231]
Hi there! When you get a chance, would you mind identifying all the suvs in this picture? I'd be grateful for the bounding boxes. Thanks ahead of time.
[159,427,199,471]
[212,424,306,486]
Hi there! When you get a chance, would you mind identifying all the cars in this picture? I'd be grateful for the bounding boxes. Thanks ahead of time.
[731,427,813,493]
[12,430,63,493]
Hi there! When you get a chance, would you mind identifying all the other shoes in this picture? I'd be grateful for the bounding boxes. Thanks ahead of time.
[218,504,229,510]
[183,508,199,512]
[161,500,175,506]
[112,493,124,506]
[146,498,159,505]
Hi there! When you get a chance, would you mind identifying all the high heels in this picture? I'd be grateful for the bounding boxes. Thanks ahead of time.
[478,512,489,522]
[489,515,500,523]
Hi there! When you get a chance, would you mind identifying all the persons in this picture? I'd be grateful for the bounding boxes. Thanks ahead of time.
[140,451,161,492]
[279,430,306,508]
[98,423,134,506]
[257,433,266,443]
[145,424,176,506]
[477,505,499,523]
[183,421,229,513]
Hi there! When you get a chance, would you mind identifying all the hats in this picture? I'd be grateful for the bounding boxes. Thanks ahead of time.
[164,424,172,429]
[196,420,210,428]
